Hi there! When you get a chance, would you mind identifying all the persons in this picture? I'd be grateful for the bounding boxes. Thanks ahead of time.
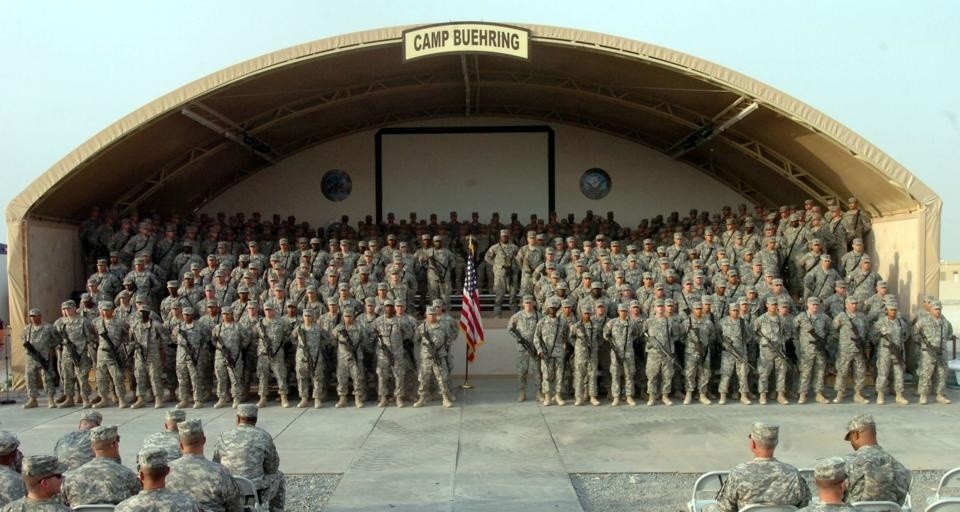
[212,402,287,511]
[699,419,812,511]
[58,423,143,506]
[163,417,246,511]
[0,428,26,506]
[136,408,186,470]
[1,454,75,510]
[842,412,912,507]
[112,446,202,511]
[52,410,124,470]
[793,454,861,511]
[19,195,955,408]
[14,449,24,473]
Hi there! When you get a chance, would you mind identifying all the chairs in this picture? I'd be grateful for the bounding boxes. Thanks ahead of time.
[232,474,269,512]
[687,467,960,512]
[73,504,117,511]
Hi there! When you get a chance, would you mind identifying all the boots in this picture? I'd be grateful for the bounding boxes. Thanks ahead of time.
[798,393,810,403]
[895,392,908,404]
[853,391,869,403]
[538,389,768,406]
[517,391,525,402]
[257,391,455,408]
[833,391,844,403]
[936,394,952,403]
[876,391,885,404]
[777,391,790,405]
[816,391,830,403]
[22,392,241,409]
[919,394,929,403]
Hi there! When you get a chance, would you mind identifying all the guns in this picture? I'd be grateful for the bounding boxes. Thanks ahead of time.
[503,266,513,285]
[403,339,417,371]
[809,329,833,362]
[99,332,124,369]
[785,339,800,373]
[606,334,622,363]
[216,336,236,368]
[716,333,756,370]
[298,329,315,376]
[132,332,146,363]
[179,330,197,368]
[340,329,358,361]
[373,328,394,366]
[430,255,445,283]
[883,334,905,365]
[60,331,81,367]
[23,341,59,386]
[511,323,533,353]
[756,329,784,359]
[919,329,947,366]
[454,239,468,266]
[645,335,683,370]
[423,331,441,365]
[851,326,867,359]
[260,324,275,358]
[539,336,548,354]
[692,328,704,376]
[578,324,593,358]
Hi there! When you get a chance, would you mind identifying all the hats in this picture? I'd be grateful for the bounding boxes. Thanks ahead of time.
[62,205,159,312]
[813,457,849,480]
[748,422,780,440]
[28,308,40,316]
[885,302,898,308]
[808,297,821,305]
[0,432,20,455]
[167,212,457,316]
[851,238,865,246]
[237,403,258,417]
[522,201,783,309]
[472,211,518,235]
[780,198,861,222]
[138,446,167,468]
[22,454,69,475]
[844,296,859,304]
[844,413,875,441]
[811,238,821,245]
[820,254,831,260]
[877,281,888,287]
[834,280,846,287]
[80,409,102,425]
[88,425,119,440]
[523,198,899,310]
[861,256,872,263]
[166,409,203,434]
[924,296,942,310]
